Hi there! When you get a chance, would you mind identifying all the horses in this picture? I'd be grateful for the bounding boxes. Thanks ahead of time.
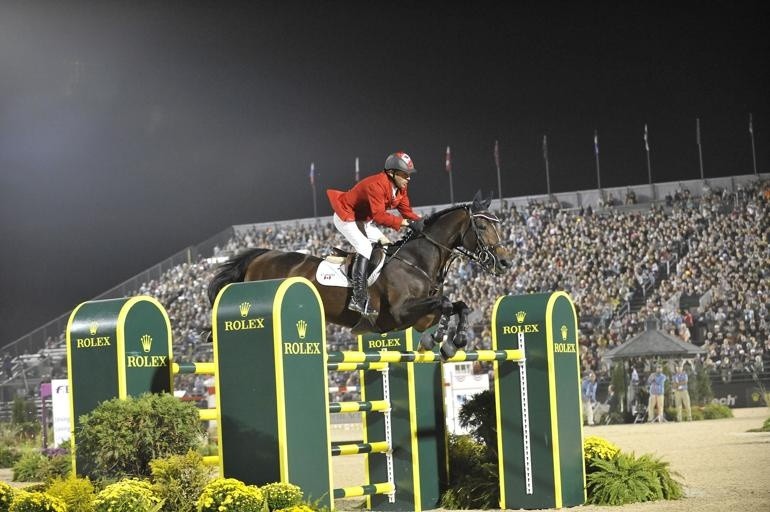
[208,187,514,360]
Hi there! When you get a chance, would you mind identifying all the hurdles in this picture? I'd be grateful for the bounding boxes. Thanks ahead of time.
[170,363,222,467]
[326,362,394,501]
[322,351,523,362]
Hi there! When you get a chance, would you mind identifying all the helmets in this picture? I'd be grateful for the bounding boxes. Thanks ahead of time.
[383,150,418,174]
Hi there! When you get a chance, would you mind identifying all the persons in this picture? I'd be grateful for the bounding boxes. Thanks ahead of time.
[582,372,596,423]
[592,385,619,423]
[326,153,419,317]
[420,171,769,382]
[647,363,666,422]
[670,367,692,422]
[0,213,362,408]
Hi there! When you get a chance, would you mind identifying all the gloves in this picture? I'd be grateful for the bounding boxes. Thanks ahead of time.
[408,217,429,233]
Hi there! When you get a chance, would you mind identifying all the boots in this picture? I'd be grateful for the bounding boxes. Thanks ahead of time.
[347,252,381,317]
